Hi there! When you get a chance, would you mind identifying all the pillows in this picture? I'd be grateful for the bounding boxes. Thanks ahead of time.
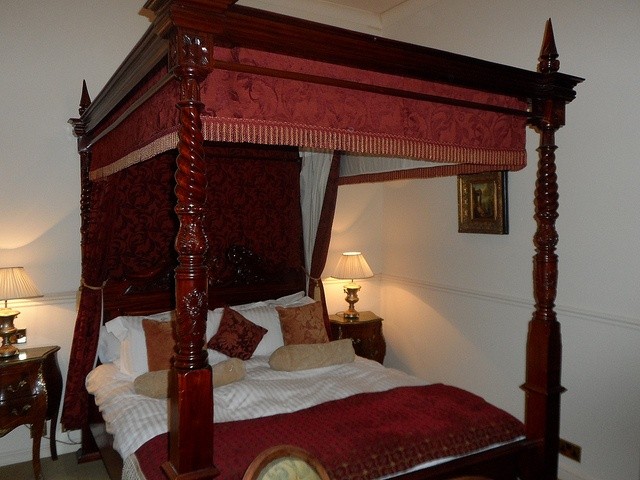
[206,307,268,361]
[269,339,356,371]
[105,311,226,379]
[275,301,330,346]
[214,292,316,362]
[134,357,246,400]
[141,317,209,371]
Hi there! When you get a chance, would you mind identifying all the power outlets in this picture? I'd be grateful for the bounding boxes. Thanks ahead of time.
[556,437,582,463]
[14,329,27,344]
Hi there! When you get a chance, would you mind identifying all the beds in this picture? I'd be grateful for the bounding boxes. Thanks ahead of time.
[76,237,567,480]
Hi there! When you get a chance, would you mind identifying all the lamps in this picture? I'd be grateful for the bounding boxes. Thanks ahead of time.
[0,266,44,358]
[330,252,374,318]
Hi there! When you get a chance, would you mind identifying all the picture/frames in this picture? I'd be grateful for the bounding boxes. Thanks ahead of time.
[456,171,510,235]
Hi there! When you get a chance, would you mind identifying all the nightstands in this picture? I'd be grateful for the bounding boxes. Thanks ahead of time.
[0,345,63,480]
[327,311,386,365]
